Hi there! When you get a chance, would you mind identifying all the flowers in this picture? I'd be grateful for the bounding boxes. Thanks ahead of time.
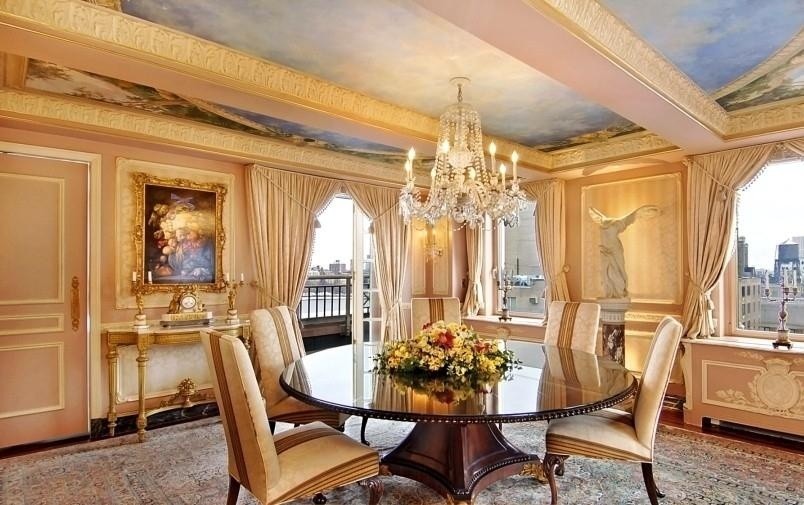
[386,374,497,407]
[370,319,522,380]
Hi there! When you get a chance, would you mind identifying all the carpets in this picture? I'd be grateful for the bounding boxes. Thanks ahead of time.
[0,412,804,503]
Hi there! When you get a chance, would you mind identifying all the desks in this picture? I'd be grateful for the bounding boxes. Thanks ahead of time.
[275,337,639,504]
[95,314,258,442]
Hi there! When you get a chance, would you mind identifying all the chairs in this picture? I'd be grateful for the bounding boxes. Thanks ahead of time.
[535,298,602,426]
[545,345,601,389]
[409,295,504,436]
[537,316,686,505]
[195,327,384,504]
[250,305,355,504]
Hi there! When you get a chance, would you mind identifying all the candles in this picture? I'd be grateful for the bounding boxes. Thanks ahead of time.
[224,272,229,282]
[147,270,153,283]
[240,272,244,281]
[516,256,520,276]
[495,268,514,287]
[761,264,798,294]
[131,271,137,283]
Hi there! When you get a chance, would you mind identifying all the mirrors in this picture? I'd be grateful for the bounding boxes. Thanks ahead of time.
[491,192,547,321]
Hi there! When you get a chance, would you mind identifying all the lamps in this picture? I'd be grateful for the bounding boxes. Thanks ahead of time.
[396,76,528,232]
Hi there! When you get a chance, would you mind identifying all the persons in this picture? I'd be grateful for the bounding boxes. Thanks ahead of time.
[588,205,637,297]
[152,211,215,281]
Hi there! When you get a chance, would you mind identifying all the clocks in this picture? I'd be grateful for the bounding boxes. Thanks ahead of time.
[167,283,203,314]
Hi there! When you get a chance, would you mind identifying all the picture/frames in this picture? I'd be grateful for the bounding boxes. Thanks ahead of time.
[134,171,228,296]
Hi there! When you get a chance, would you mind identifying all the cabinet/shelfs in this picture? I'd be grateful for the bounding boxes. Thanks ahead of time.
[673,334,804,453]
